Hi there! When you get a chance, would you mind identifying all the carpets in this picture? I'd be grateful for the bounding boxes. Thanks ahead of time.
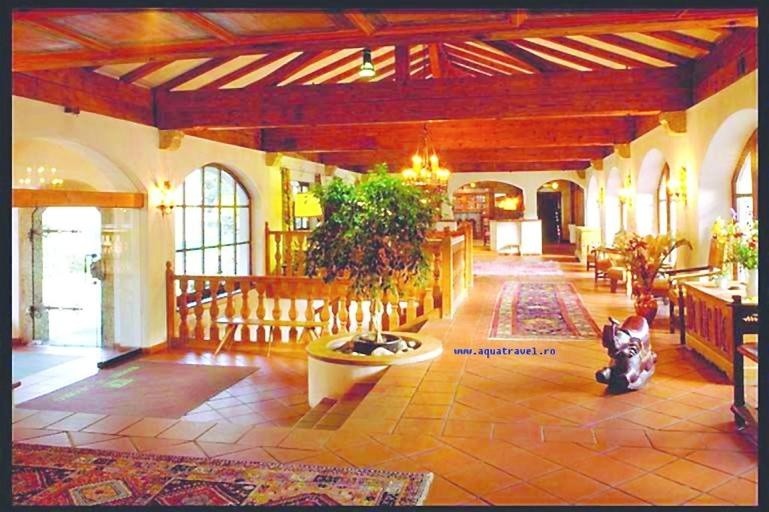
[14,359,261,421]
[11,440,434,506]
[488,281,603,342]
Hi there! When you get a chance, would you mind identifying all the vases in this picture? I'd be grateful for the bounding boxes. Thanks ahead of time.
[714,270,759,297]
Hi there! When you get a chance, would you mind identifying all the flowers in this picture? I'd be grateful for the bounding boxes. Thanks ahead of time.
[709,202,758,275]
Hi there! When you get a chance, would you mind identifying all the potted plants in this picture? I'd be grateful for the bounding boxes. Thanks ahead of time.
[289,162,456,356]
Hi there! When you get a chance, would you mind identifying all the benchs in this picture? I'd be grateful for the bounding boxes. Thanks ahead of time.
[213,317,330,358]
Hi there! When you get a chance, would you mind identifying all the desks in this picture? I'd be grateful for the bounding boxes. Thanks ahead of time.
[680,280,759,385]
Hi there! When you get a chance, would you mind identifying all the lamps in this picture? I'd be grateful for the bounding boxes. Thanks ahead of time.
[358,48,375,78]
